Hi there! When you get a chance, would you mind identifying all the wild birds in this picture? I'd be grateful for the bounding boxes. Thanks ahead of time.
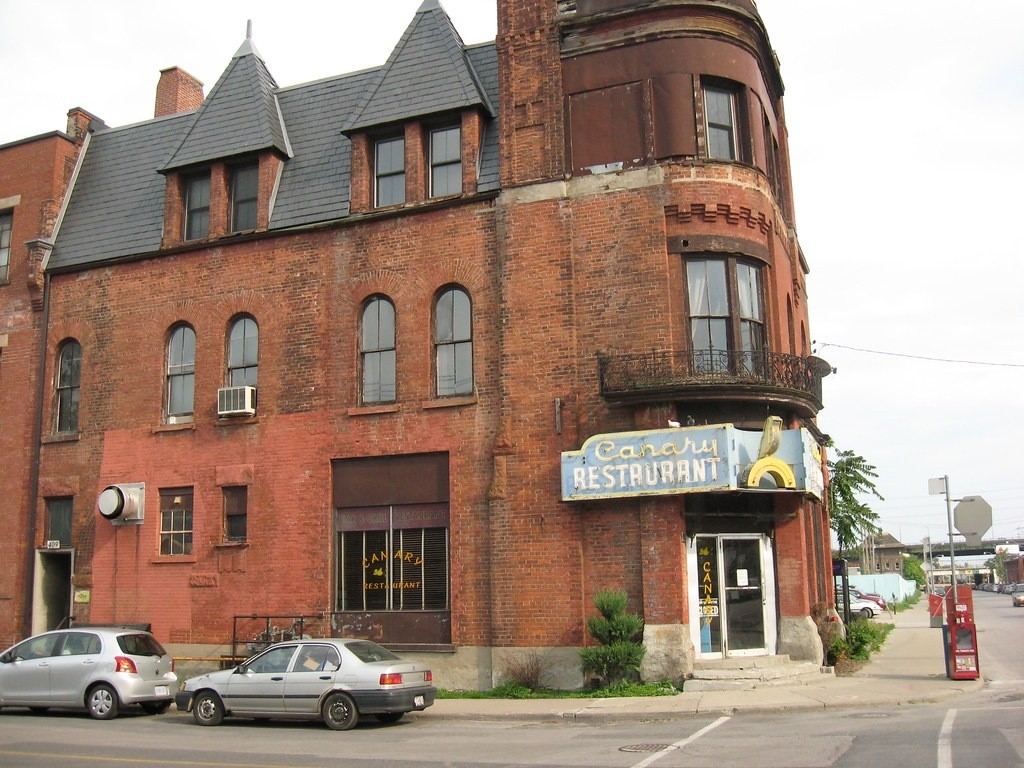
[668,415,695,428]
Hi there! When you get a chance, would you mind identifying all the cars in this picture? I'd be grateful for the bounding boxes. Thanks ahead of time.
[0,628,179,720]
[1012,584,1024,607]
[175,637,436,730]
[832,585,886,619]
[972,583,1013,594]
[935,586,946,596]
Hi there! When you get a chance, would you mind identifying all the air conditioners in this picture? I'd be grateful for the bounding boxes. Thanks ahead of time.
[217,386,256,415]
